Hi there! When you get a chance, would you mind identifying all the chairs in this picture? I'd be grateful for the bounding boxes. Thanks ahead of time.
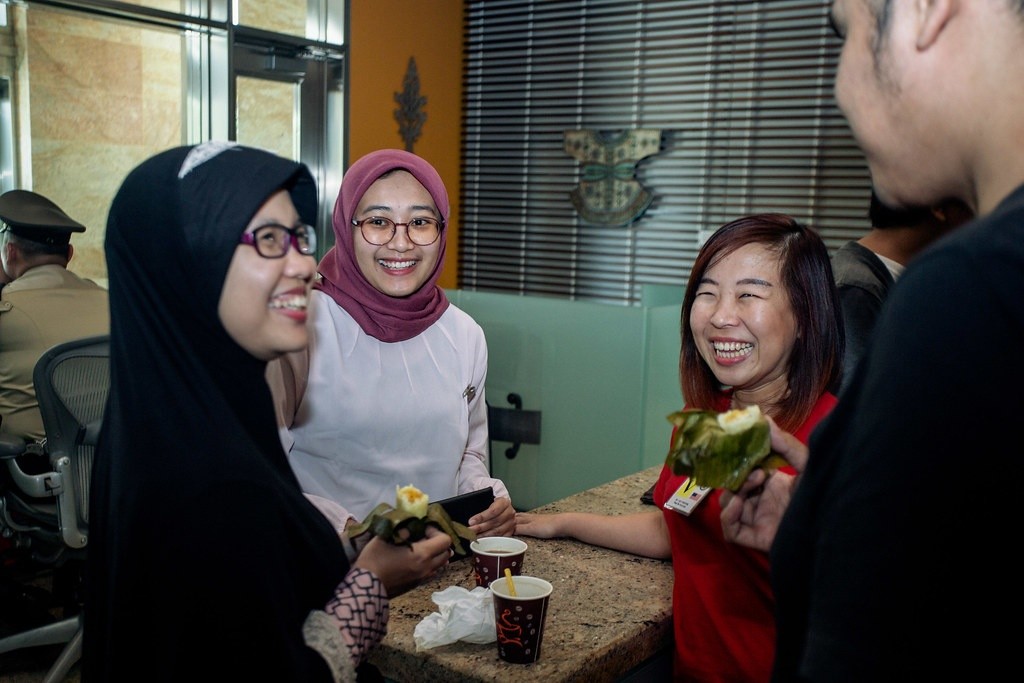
[0,334,110,683]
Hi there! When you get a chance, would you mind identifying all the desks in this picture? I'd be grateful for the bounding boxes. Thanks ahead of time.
[363,463,675,683]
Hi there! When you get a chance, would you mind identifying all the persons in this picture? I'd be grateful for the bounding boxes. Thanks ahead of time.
[718,1,1024,683]
[267,149,516,562]
[513,212,846,683]
[80,140,451,683]
[826,188,972,397]
[0,189,110,501]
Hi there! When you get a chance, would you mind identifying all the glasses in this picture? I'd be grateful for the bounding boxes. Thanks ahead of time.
[352,215,447,246]
[238,223,318,258]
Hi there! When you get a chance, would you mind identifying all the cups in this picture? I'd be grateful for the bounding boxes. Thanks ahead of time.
[490,576,553,664]
[469,537,528,588]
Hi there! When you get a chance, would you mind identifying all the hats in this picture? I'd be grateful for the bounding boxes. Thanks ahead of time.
[0,189,87,244]
